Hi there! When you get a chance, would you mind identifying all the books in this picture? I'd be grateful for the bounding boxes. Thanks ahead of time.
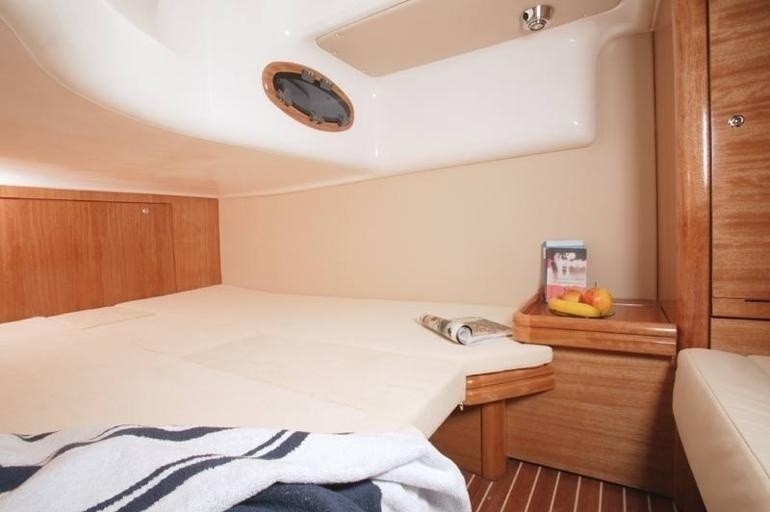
[419,312,512,346]
[544,246,588,304]
[541,240,585,302]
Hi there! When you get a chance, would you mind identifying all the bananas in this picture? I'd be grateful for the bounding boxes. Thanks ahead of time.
[548,297,601,318]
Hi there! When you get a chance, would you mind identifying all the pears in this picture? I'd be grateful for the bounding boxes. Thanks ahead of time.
[561,287,581,303]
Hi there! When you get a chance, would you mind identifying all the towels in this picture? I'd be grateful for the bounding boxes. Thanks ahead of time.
[0,424,474,512]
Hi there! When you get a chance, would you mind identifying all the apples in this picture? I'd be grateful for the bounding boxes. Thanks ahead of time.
[583,280,612,316]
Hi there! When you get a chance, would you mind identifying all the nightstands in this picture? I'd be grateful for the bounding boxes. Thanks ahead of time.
[508,288,679,497]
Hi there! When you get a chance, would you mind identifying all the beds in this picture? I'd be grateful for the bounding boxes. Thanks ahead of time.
[0,186,554,512]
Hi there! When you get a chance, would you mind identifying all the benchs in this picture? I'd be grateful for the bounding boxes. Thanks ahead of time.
[671,348,770,512]
